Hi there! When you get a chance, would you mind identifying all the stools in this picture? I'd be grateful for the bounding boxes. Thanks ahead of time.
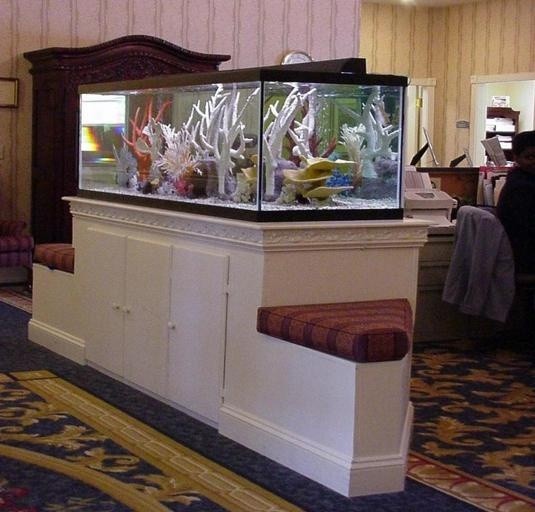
[255,299,414,498]
[25,241,83,367]
[0,219,33,285]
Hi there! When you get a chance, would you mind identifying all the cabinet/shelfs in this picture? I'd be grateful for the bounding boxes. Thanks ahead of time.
[80,226,172,402]
[20,32,232,297]
[169,245,230,433]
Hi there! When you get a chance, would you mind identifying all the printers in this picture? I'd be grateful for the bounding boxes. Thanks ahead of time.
[402,164,458,223]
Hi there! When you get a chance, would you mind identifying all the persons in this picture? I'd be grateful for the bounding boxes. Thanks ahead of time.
[495,130,535,276]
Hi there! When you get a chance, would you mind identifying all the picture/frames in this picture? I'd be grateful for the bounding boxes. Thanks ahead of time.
[0,76,20,110]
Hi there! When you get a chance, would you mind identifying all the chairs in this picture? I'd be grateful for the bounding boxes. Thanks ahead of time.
[452,202,534,341]
